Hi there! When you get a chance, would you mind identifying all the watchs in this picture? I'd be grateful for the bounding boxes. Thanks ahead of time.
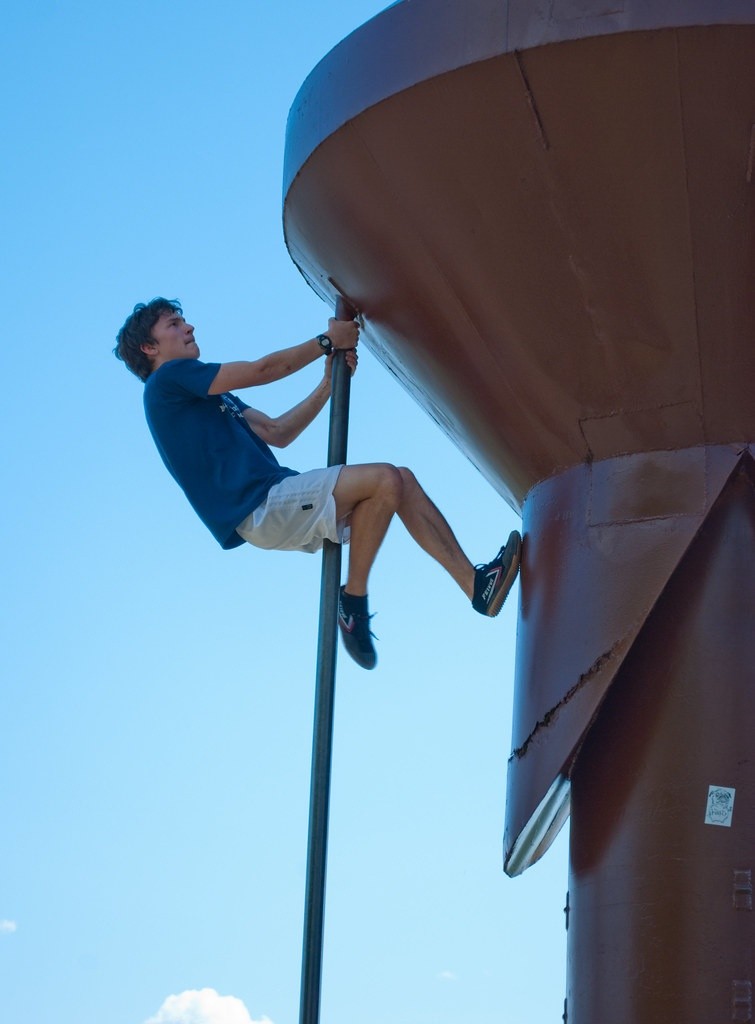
[315,334,333,356]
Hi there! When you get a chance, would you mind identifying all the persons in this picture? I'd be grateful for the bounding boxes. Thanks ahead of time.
[112,295,523,671]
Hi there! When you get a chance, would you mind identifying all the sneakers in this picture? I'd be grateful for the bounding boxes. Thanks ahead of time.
[338,585,380,671]
[472,530,521,618]
